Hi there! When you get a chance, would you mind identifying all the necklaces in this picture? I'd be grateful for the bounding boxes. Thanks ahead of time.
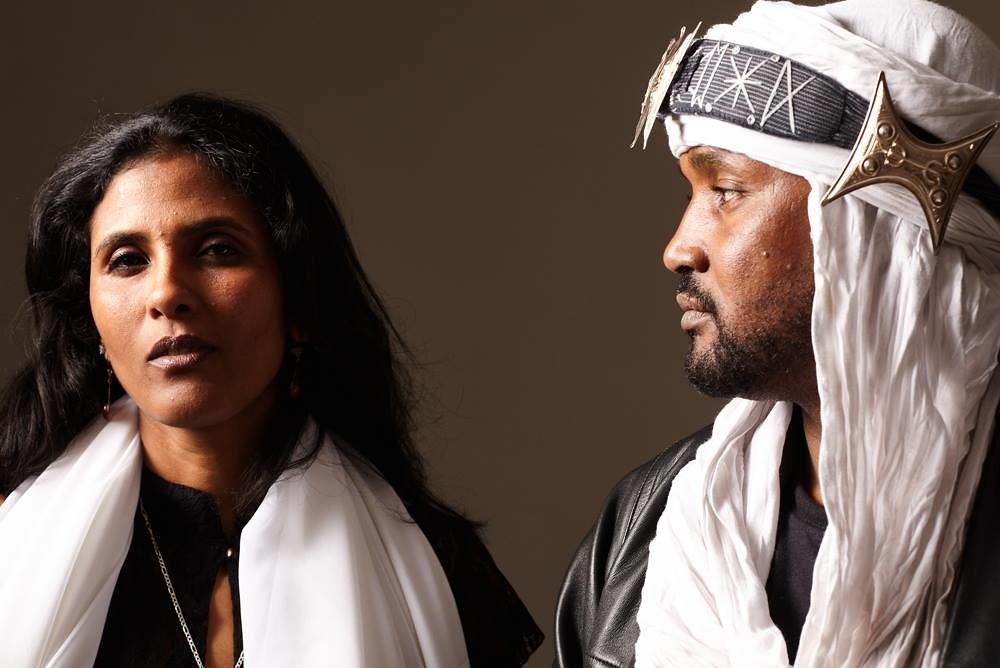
[137,501,246,668]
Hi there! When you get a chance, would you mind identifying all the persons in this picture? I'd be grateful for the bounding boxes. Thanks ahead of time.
[0,91,544,668]
[548,0,1000,668]
